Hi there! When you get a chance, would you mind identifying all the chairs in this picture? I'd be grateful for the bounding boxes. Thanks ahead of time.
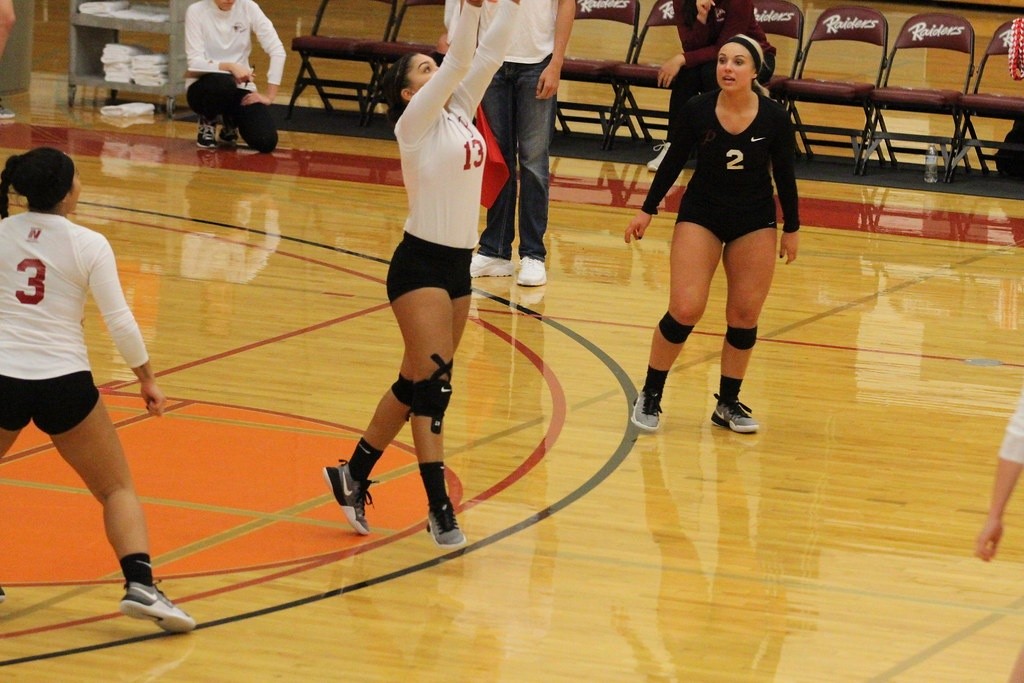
[946,17,1024,183]
[784,5,889,176]
[860,12,975,180]
[286,0,804,157]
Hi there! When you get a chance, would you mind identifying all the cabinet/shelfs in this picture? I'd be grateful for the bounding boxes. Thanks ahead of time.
[67,0,201,119]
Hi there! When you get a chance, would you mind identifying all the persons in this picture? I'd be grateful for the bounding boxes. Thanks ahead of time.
[323,1,520,540]
[0,146,195,635]
[184,0,287,154]
[974,393,1024,682]
[625,32,801,434]
[646,0,778,171]
[444,1,574,286]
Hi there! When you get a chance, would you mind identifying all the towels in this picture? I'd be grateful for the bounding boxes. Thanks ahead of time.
[100,119,155,128]
[100,101,156,118]
[132,53,170,87]
[101,44,152,83]
[78,1,169,22]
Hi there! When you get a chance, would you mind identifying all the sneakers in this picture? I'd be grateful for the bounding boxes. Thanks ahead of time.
[218,124,239,143]
[516,256,546,286]
[470,255,513,276]
[120,582,195,633]
[509,285,545,312]
[196,149,218,170]
[647,138,671,170]
[470,279,511,299]
[322,458,380,536]
[630,388,661,430]
[196,118,217,147]
[425,496,466,549]
[710,393,760,433]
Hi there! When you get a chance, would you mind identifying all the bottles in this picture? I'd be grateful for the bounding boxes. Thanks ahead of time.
[924,143,939,184]
